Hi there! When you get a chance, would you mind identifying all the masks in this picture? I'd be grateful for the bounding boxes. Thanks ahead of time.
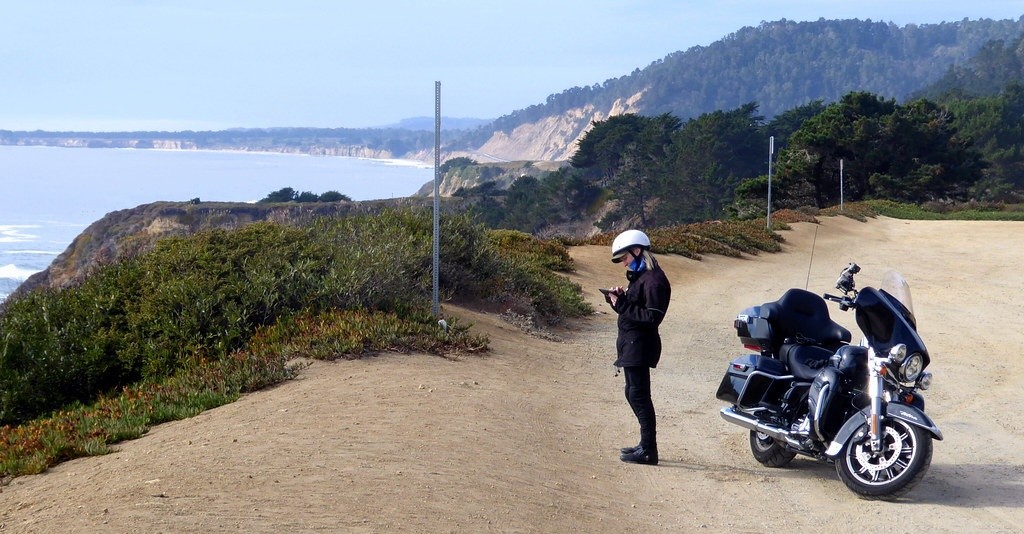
[629,255,646,272]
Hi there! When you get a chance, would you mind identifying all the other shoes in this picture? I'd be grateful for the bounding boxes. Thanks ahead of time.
[620,448,658,465]
[621,443,642,454]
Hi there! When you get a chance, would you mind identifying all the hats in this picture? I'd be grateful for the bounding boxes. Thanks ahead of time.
[611,246,634,263]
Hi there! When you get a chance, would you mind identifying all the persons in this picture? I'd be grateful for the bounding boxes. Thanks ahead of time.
[604,229,672,465]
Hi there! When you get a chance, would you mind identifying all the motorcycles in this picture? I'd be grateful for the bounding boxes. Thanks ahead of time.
[713,263,946,497]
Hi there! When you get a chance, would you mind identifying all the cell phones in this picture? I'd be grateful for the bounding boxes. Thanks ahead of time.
[599,288,617,297]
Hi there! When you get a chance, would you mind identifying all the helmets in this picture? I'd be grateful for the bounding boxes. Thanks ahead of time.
[612,230,650,255]
